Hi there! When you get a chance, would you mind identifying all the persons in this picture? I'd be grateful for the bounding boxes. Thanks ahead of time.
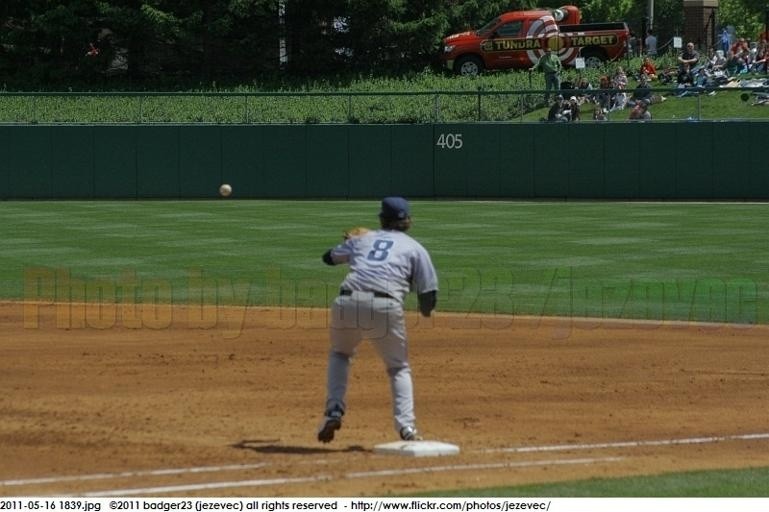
[527,22,769,123]
[318,196,440,445]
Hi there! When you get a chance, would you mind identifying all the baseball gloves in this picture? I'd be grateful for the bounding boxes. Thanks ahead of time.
[346,227,367,239]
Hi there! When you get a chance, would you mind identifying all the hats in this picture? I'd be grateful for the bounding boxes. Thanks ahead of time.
[556,94,577,102]
[378,197,410,219]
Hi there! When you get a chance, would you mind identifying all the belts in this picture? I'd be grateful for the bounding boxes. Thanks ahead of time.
[340,290,393,298]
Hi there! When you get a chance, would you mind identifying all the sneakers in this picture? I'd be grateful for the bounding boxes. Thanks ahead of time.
[399,424,417,441]
[317,408,343,444]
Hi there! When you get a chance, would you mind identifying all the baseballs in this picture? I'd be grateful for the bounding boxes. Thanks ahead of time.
[220,184,232,194]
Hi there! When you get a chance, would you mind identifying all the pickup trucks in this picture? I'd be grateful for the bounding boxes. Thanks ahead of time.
[440,5,632,77]
